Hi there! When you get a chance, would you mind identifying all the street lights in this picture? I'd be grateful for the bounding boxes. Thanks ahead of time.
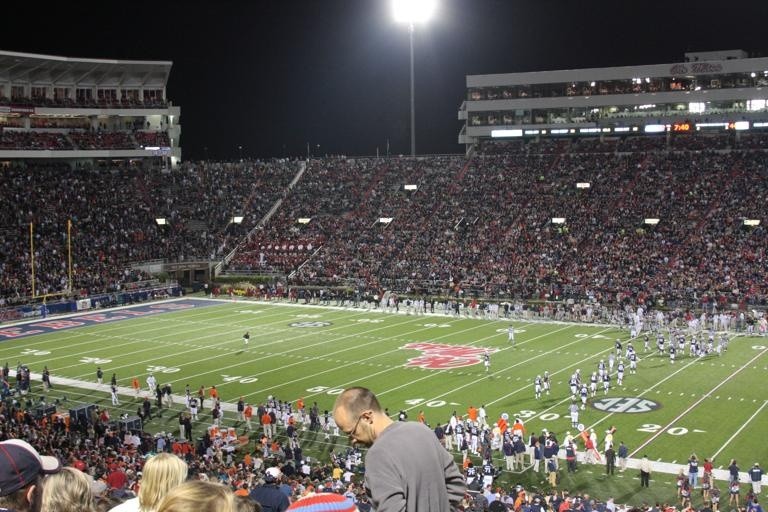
[388,0,443,157]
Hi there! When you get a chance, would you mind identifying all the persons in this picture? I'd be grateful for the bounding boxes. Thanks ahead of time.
[0,75,768,512]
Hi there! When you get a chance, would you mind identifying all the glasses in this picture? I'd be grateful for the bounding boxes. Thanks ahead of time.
[338,416,361,438]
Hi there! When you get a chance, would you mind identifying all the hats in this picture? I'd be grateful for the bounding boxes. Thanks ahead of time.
[285,490,359,512]
[261,465,282,483]
[0,438,61,497]
